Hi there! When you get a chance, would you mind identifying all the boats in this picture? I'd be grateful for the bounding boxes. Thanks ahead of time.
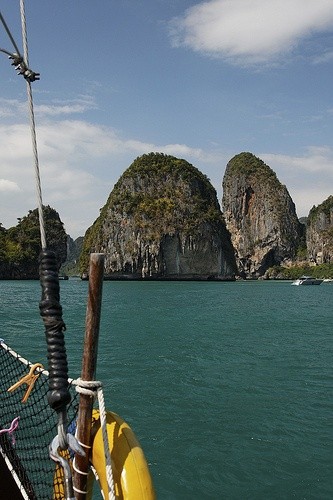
[80,272,89,280]
[58,276,68,280]
[291,276,324,285]
[316,277,333,283]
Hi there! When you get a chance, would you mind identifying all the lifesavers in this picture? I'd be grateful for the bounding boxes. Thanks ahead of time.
[54,407,155,500]
